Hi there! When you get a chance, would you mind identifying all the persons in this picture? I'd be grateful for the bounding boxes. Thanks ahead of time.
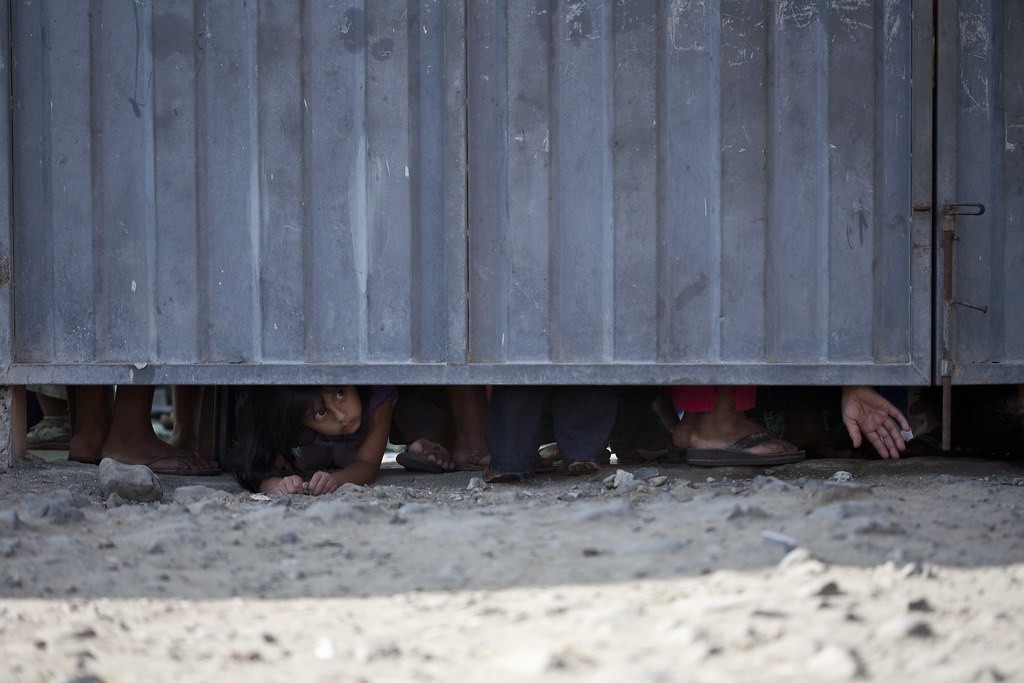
[451,385,1024,485]
[68,385,224,475]
[24,384,73,443]
[233,385,450,494]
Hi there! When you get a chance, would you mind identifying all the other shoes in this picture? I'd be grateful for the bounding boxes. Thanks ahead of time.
[26,420,72,449]
[483,465,520,482]
[568,458,601,475]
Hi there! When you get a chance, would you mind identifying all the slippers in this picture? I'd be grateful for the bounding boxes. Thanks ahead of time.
[687,430,805,466]
[534,455,553,473]
[396,438,453,471]
[455,446,492,470]
[141,452,221,475]
